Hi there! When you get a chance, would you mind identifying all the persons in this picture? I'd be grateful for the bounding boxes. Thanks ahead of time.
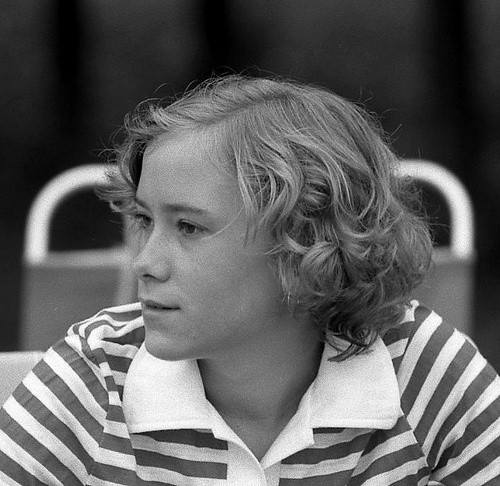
[0,72,500,486]
